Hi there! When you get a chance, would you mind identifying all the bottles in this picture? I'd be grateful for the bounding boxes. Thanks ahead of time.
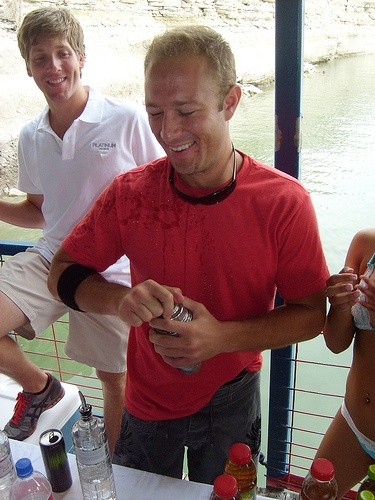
[225,443,258,500]
[0,429,18,500]
[72,390,118,500]
[151,302,202,374]
[356,464,375,496]
[9,457,55,500]
[357,489,375,500]
[209,475,243,500]
[298,457,338,500]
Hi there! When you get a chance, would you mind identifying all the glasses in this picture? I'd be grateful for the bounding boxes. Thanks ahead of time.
[167,140,237,206]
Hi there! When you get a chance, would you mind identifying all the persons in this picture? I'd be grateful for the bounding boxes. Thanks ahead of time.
[47,26,329,486]
[0,6,163,465]
[309,226,375,499]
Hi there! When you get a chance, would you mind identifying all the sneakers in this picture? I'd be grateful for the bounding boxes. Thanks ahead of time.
[5,371,65,441]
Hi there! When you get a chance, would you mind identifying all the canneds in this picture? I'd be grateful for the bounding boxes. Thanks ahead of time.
[39,429,72,493]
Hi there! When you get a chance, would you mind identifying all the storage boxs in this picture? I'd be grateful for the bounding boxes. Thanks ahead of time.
[0,371,85,454]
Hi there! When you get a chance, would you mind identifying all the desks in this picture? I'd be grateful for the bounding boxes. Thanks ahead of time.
[9,438,280,500]
